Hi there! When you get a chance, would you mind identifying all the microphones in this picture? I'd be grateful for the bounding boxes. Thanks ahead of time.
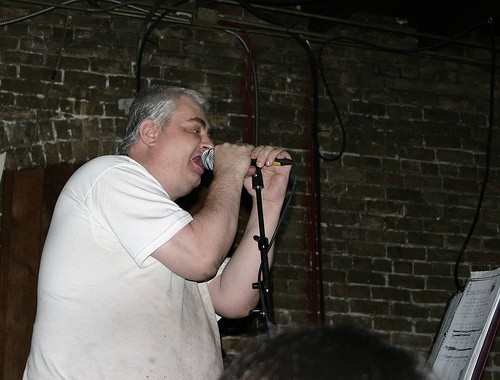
[201,148,293,172]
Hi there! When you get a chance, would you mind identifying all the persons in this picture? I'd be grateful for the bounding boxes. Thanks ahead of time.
[21,85,293,380]
